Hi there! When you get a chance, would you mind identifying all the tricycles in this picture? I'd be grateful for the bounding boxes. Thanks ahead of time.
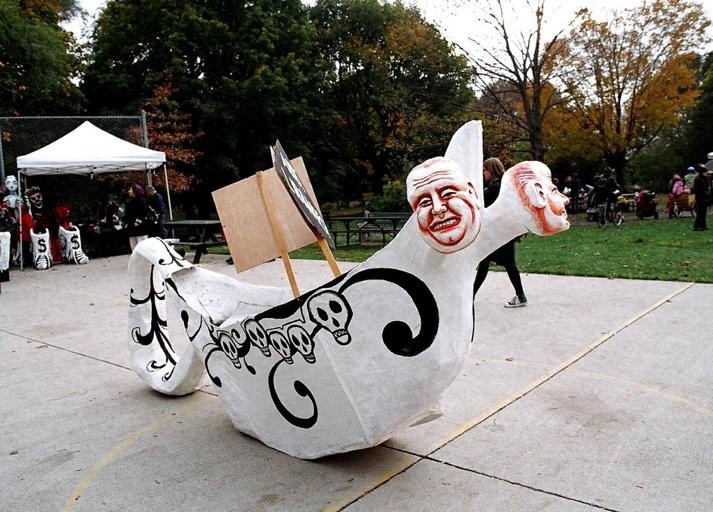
[673,191,696,220]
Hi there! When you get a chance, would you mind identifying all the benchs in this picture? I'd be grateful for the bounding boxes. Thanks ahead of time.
[563,189,696,217]
[161,242,214,264]
[331,216,405,247]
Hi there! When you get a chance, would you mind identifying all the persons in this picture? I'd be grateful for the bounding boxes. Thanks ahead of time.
[472,157,527,307]
[502,160,571,238]
[550,150,712,232]
[91,182,166,257]
[14,204,34,269]
[406,156,483,255]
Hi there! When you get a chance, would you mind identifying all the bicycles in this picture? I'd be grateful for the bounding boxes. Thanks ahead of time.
[594,198,624,229]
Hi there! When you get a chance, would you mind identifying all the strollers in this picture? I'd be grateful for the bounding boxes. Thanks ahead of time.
[630,189,659,220]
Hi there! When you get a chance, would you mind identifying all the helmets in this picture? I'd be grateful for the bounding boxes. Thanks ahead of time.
[687,166,694,173]
[695,163,706,173]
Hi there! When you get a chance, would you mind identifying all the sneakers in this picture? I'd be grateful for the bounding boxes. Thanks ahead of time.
[503,293,527,308]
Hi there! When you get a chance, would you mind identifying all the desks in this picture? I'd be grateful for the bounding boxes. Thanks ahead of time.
[164,217,222,266]
